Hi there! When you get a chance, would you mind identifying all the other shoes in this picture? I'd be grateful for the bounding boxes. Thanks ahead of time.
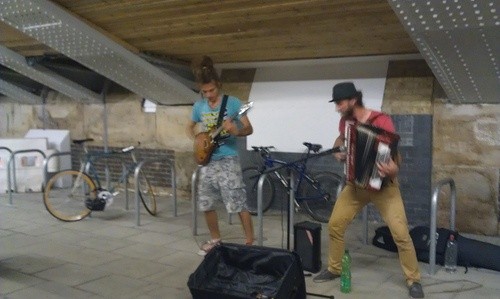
[313,269,340,283]
[198,240,220,255]
[409,282,424,299]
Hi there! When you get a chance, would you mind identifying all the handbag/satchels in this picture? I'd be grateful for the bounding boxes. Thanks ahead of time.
[372,226,500,271]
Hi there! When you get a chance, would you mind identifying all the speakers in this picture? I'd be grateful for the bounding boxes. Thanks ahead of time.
[187,242,306,299]
[294,221,322,273]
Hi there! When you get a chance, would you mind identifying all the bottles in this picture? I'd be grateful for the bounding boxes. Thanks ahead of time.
[444,235,457,272]
[340,251,351,293]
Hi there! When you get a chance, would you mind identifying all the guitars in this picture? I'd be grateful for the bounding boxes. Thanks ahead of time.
[193,102,253,166]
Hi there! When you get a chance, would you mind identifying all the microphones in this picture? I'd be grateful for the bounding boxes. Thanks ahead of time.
[320,146,346,156]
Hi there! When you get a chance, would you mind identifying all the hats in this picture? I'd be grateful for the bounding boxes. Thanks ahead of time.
[328,82,358,102]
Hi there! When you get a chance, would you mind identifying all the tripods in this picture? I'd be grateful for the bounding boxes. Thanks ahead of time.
[247,154,334,299]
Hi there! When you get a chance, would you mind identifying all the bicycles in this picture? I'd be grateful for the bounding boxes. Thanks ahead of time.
[42,137,158,223]
[241,142,346,224]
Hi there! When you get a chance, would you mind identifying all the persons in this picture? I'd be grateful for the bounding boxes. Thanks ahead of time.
[313,82,424,299]
[189,55,254,255]
